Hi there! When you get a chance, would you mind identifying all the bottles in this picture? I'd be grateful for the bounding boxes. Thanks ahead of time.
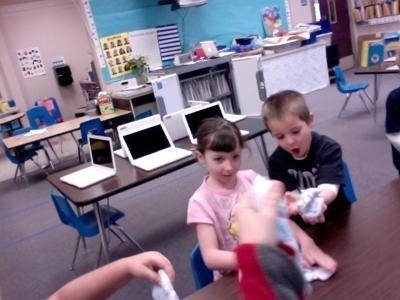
[98,91,115,115]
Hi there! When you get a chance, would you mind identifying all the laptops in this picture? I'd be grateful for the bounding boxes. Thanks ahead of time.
[59,134,117,190]
[117,119,193,172]
[162,104,202,142]
[113,113,161,159]
[187,100,247,124]
[182,100,250,145]
[200,41,235,59]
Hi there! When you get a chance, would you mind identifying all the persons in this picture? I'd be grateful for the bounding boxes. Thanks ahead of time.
[186,117,286,280]
[103,38,133,74]
[46,250,177,300]
[232,177,304,300]
[259,90,356,229]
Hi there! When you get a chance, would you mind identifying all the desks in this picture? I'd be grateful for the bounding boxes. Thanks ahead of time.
[179,175,400,300]
[46,116,270,264]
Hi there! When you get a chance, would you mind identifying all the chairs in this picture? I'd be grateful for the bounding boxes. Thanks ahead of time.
[188,243,214,290]
[0,97,154,271]
[332,65,376,118]
[339,160,358,204]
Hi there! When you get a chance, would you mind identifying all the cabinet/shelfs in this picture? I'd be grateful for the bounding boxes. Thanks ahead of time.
[110,38,330,118]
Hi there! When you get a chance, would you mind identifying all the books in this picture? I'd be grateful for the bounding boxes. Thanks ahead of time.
[181,73,236,115]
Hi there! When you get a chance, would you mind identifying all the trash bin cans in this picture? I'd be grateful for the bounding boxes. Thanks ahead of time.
[326,44,341,84]
